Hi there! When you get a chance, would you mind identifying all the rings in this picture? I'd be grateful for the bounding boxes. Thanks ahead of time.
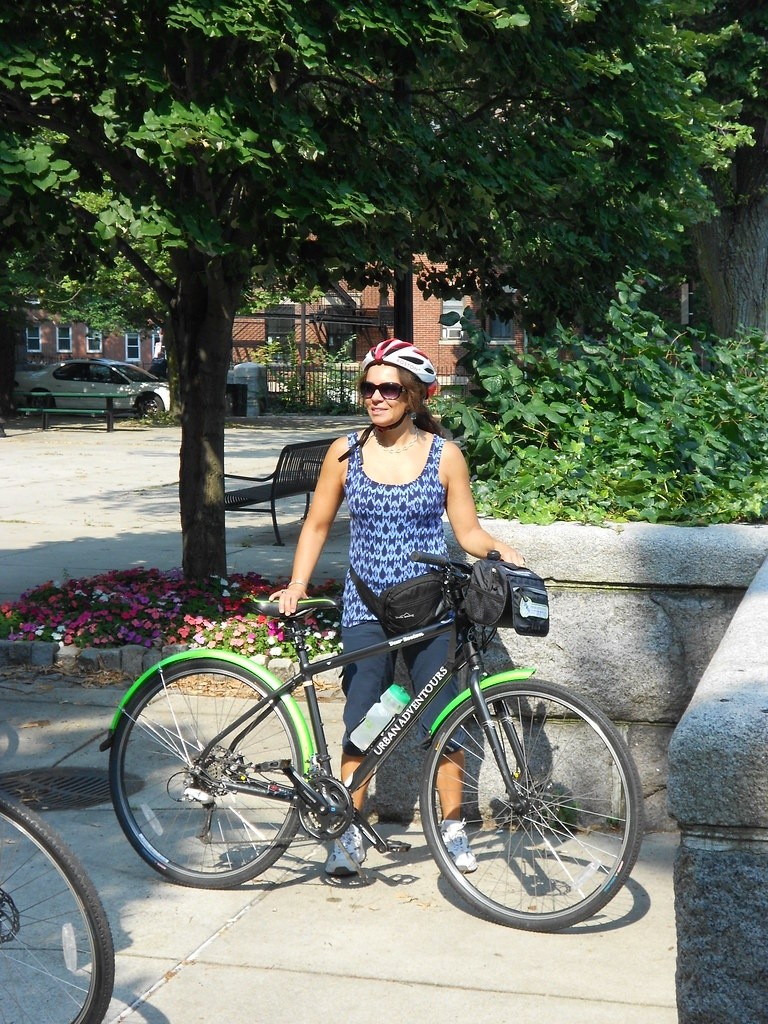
[521,562,525,565]
[281,590,285,594]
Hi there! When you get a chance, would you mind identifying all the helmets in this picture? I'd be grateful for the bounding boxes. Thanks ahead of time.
[361,339,440,401]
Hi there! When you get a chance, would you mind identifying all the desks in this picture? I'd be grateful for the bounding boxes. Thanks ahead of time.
[16,392,138,432]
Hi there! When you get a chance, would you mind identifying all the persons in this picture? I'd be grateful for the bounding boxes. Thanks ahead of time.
[268,338,525,873]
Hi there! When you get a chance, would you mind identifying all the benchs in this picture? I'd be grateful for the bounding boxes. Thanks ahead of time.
[18,408,109,414]
[113,408,137,413]
[223,437,339,547]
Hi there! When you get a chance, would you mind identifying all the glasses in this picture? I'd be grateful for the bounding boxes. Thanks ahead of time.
[359,381,407,401]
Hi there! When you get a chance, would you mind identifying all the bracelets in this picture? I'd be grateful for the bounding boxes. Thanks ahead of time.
[289,581,306,588]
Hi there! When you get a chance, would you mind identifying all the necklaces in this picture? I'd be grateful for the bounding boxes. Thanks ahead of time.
[374,425,418,454]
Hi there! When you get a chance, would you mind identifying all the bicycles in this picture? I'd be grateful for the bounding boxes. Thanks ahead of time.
[99,551,647,933]
[0,791,118,1024]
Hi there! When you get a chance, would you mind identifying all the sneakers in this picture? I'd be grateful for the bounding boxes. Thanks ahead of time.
[325,828,367,877]
[439,817,478,873]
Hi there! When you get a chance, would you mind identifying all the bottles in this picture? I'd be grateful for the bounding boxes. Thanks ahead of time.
[347,684,411,752]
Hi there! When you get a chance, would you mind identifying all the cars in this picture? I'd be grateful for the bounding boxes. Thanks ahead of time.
[11,357,171,419]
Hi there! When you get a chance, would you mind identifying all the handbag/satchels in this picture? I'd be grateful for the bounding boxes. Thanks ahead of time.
[348,562,448,638]
